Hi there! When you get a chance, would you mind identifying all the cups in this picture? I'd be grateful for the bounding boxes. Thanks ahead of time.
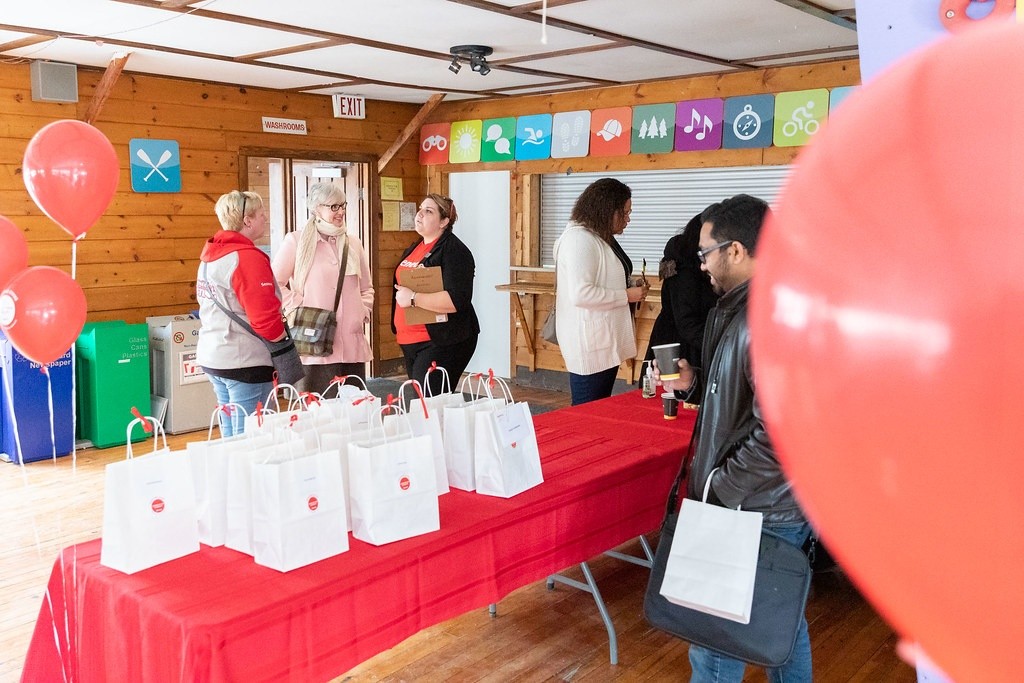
[661,392,680,419]
[651,343,681,380]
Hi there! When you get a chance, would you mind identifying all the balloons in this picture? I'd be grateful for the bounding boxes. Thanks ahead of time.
[0,215,28,292]
[23,120,120,237]
[0,266,88,365]
[749,10,1024,683]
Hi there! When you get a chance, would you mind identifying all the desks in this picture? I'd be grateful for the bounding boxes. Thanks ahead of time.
[20,389,699,683]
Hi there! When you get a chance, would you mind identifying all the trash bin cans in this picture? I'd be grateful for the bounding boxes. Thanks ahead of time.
[0,326,77,465]
[76,320,153,448]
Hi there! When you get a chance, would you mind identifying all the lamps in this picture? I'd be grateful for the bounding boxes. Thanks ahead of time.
[448,45,494,76]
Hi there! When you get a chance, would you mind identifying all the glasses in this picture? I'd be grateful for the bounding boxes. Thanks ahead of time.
[443,196,454,222]
[320,202,347,212]
[238,190,251,225]
[697,239,738,265]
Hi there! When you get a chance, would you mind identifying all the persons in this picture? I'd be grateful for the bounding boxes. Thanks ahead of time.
[389,194,481,399]
[652,195,819,683]
[271,181,375,399]
[638,214,720,392]
[553,179,650,406]
[193,191,288,438]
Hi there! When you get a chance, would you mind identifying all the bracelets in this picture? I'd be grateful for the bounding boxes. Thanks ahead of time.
[410,292,416,307]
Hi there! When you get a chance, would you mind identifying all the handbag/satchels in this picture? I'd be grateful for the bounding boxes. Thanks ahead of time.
[264,328,305,389]
[658,470,766,624]
[540,312,560,346]
[287,305,337,356]
[642,492,810,666]
[100,361,545,575]
